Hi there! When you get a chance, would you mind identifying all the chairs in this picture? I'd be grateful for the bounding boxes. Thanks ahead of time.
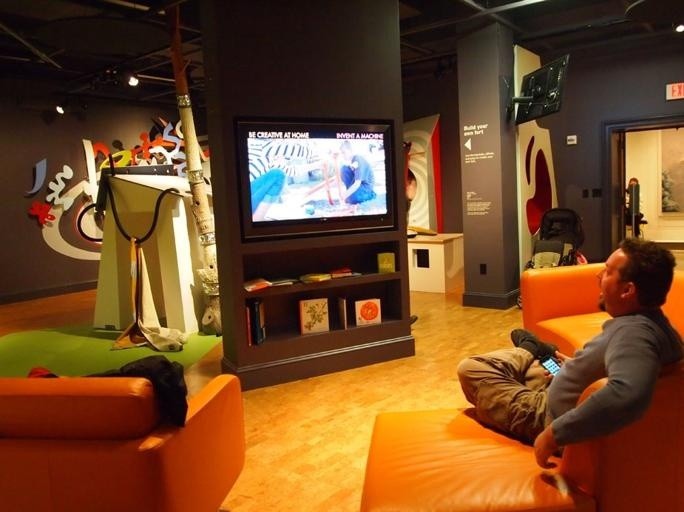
[0,369,251,506]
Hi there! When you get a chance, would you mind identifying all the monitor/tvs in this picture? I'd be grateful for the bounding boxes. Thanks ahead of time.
[232,115,399,244]
[515,53,571,127]
[94,164,175,208]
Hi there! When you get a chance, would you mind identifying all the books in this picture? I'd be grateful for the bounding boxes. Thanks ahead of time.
[245,297,266,346]
[299,267,353,284]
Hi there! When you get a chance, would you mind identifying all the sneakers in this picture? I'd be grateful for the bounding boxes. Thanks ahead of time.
[510,328,561,362]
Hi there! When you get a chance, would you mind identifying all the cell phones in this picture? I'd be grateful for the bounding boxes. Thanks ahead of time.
[539,355,561,376]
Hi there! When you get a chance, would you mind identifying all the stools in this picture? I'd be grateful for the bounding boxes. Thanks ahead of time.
[359,405,597,512]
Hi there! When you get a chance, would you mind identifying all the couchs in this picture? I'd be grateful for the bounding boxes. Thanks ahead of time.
[510,259,684,512]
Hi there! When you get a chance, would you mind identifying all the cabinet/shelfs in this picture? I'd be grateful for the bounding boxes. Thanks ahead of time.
[219,230,415,392]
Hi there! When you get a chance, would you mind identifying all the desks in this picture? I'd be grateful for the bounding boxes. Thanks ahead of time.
[407,232,460,294]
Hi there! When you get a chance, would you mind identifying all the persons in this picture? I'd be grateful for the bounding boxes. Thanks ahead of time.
[250,168,285,222]
[338,140,376,204]
[457,238,684,469]
